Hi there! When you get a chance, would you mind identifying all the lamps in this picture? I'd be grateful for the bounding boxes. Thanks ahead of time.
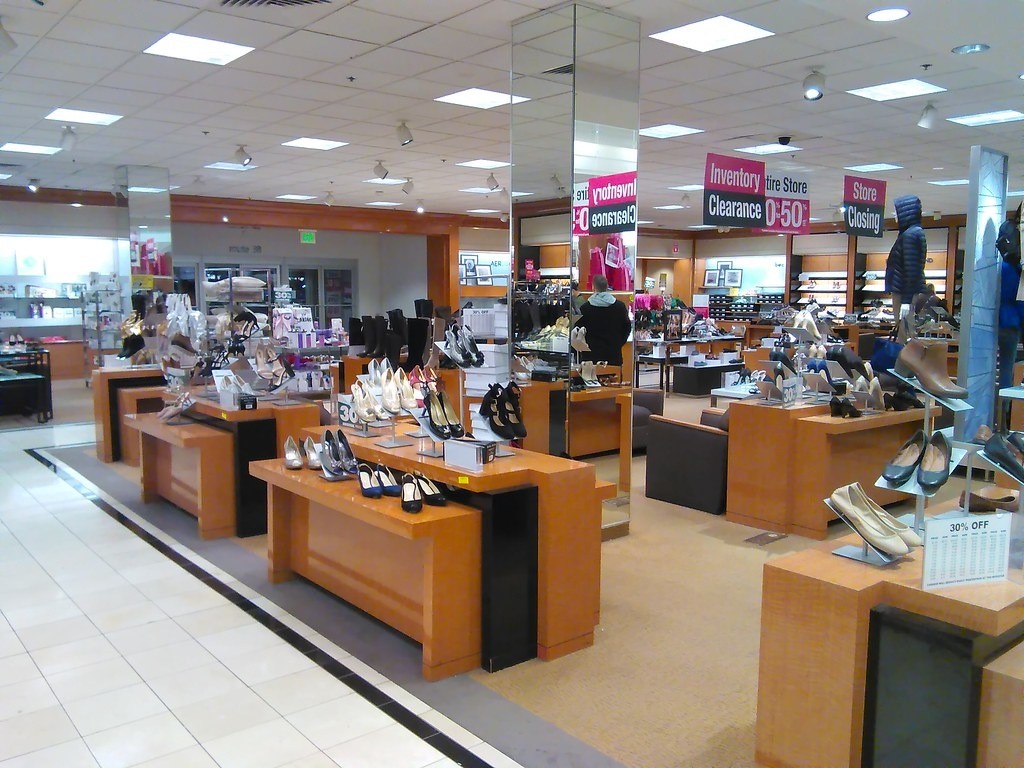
[550,176,565,190]
[802,74,825,102]
[487,176,498,191]
[59,126,79,152]
[235,149,252,167]
[324,193,335,207]
[551,188,566,199]
[0,24,18,56]
[500,213,509,222]
[402,180,414,195]
[933,212,942,220]
[28,180,40,192]
[916,104,938,129]
[396,123,413,147]
[373,162,389,179]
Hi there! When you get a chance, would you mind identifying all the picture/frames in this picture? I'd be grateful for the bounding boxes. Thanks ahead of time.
[460,254,478,276]
[716,260,733,280]
[703,269,721,287]
[474,264,494,286]
[459,264,468,286]
[724,269,743,288]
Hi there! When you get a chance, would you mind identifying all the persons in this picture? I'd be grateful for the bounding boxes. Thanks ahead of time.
[916,283,941,314]
[885,194,926,330]
[579,274,630,382]
[572,282,584,309]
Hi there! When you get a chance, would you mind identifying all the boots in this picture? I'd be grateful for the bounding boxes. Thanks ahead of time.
[894,336,969,400]
[355,297,451,373]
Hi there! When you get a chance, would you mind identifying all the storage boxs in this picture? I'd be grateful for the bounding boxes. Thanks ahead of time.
[552,336,569,354]
[719,352,738,364]
[680,346,696,355]
[531,370,558,383]
[463,303,509,442]
[239,394,258,411]
[652,344,666,356]
[287,330,316,349]
[442,439,496,464]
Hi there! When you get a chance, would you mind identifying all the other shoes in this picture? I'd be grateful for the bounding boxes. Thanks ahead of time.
[912,292,959,329]
[683,310,743,366]
[805,281,895,327]
[511,312,633,394]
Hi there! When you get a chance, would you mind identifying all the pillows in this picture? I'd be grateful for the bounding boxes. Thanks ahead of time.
[206,308,268,325]
[201,276,267,297]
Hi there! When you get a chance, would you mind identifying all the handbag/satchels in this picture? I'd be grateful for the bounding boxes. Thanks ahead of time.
[870,318,903,376]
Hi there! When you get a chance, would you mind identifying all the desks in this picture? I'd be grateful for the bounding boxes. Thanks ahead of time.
[0,371,49,424]
[672,362,745,399]
[710,383,754,408]
[0,349,54,419]
[635,352,688,390]
[634,334,746,399]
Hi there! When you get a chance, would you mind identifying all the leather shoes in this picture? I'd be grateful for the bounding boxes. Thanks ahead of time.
[829,423,1024,556]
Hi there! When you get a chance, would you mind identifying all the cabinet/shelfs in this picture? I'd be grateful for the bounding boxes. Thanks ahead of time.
[203,267,273,360]
[79,290,125,388]
[859,270,946,307]
[709,292,784,321]
[0,273,133,339]
[794,271,847,320]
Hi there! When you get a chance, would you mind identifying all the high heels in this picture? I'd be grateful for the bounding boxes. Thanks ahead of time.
[17,334,24,346]
[9,335,15,346]
[116,309,527,555]
[730,309,926,420]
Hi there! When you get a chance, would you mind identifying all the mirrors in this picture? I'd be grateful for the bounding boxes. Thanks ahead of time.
[507,1,575,457]
[567,2,643,528]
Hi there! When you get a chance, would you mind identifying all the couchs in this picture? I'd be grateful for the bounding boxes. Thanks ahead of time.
[632,387,664,455]
[645,407,728,515]
